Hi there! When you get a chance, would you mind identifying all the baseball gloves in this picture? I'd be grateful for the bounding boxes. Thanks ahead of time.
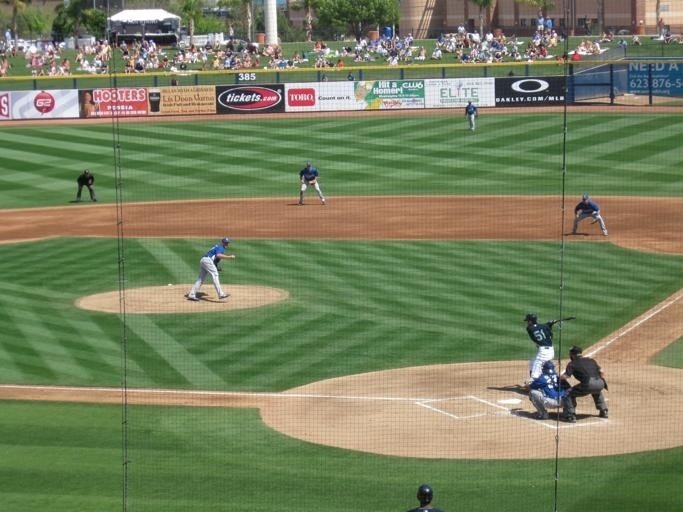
[513,382,531,393]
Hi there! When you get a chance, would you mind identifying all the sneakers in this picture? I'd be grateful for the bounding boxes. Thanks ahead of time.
[219,294,230,300]
[598,410,608,417]
[533,411,549,419]
[560,413,577,423]
[188,296,199,301]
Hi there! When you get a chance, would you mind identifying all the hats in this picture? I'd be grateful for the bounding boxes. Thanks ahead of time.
[222,238,230,243]
[583,194,588,199]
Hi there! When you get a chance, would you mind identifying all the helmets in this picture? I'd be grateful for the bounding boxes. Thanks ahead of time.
[417,484,433,503]
[84,170,89,174]
[542,361,555,374]
[570,346,582,361]
[523,314,537,322]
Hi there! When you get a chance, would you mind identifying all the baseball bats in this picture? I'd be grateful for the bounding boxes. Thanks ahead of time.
[554,317,577,323]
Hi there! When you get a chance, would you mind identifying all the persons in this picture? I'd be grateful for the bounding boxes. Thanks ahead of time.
[525,361,577,420]
[465,101,478,131]
[561,345,609,422]
[187,237,236,300]
[76,169,97,202]
[79,91,99,119]
[523,313,557,385]
[406,483,444,512]
[299,162,325,204]
[572,194,607,235]
